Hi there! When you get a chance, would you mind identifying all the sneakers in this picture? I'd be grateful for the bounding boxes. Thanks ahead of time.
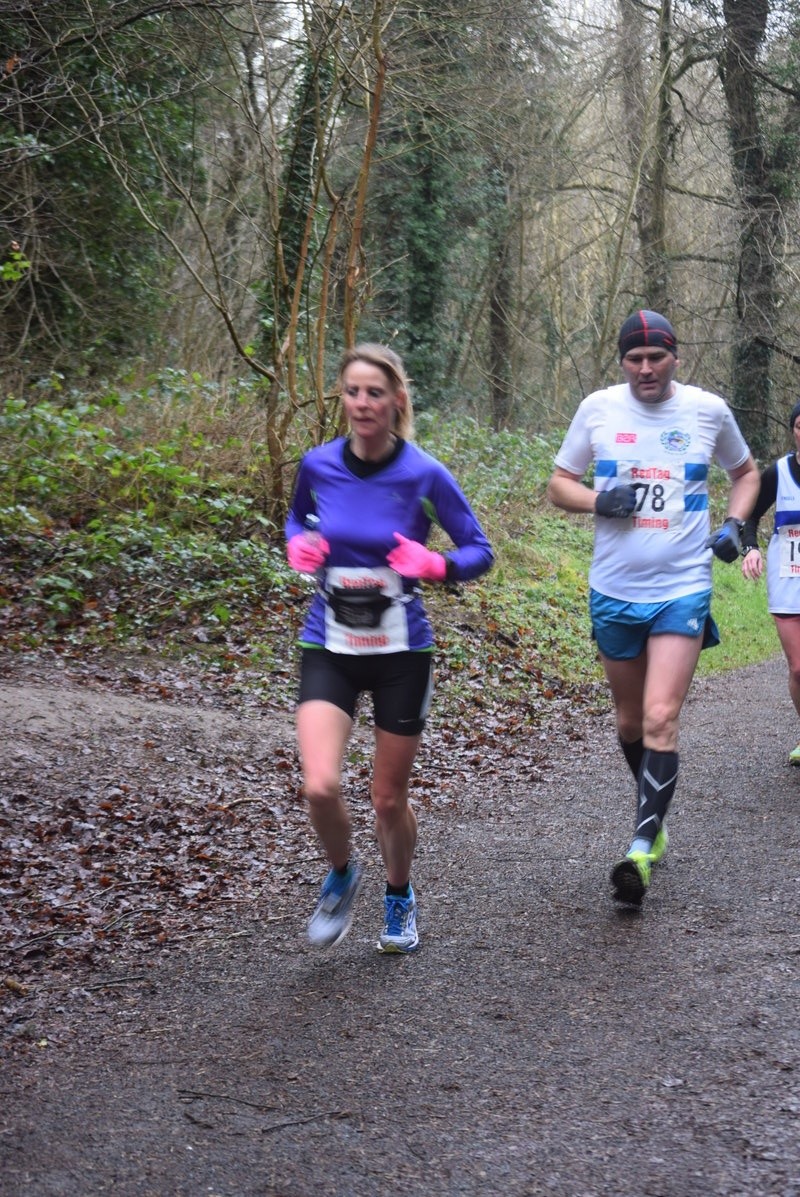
[648,817,667,863]
[788,745,800,765]
[376,878,419,954]
[308,861,363,950]
[610,850,651,911]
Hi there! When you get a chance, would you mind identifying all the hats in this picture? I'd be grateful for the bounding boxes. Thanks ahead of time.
[790,400,800,427]
[618,310,678,365]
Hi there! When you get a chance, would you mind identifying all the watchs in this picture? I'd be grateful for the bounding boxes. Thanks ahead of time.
[723,516,746,536]
[439,553,456,581]
[741,545,761,558]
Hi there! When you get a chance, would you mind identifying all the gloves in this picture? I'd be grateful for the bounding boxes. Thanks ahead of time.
[705,517,745,562]
[287,530,330,573]
[387,531,447,581]
[597,482,641,518]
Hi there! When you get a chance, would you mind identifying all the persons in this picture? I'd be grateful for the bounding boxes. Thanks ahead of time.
[740,406,800,765]
[548,310,761,911]
[284,343,498,954]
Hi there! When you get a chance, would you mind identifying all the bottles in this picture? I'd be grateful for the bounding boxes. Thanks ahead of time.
[298,513,322,595]
